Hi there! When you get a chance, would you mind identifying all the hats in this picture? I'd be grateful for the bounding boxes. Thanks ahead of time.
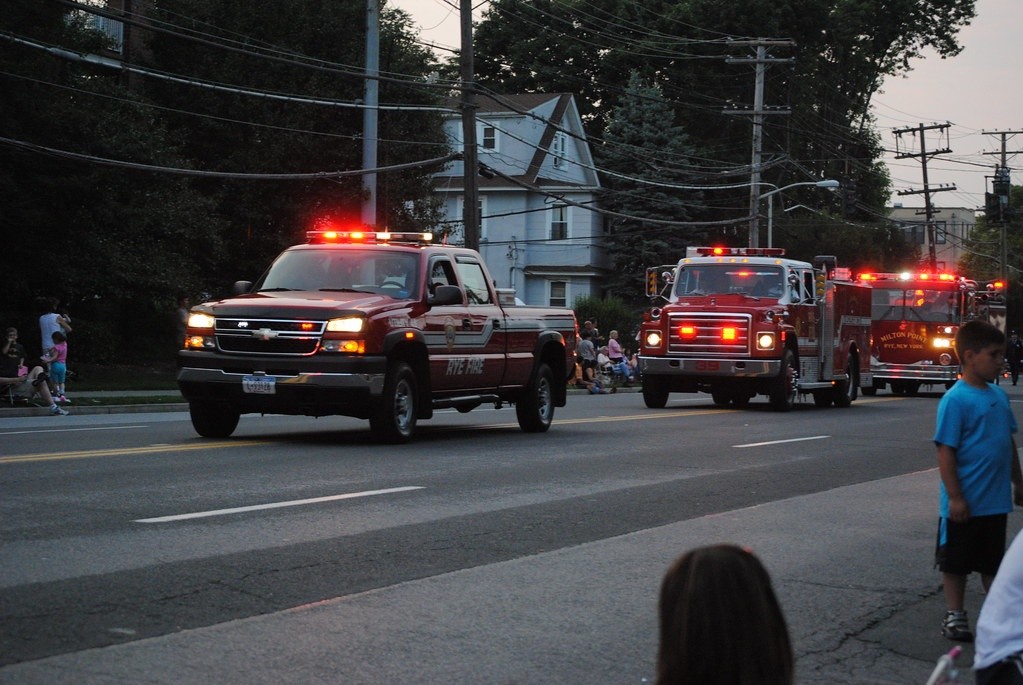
[1011,330,1018,335]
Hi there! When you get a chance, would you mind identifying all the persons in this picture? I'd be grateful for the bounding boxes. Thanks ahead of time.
[174,295,191,347]
[407,271,435,299]
[1006,329,1023,386]
[970,527,1023,685]
[768,275,800,302]
[0,294,73,416]
[651,543,795,685]
[931,322,1023,640]
[574,317,640,394]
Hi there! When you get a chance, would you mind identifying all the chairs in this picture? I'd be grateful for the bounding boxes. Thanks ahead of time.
[0,383,41,405]
[597,366,625,385]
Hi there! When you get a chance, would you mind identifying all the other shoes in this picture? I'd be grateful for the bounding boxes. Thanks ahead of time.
[51,389,55,394]
[629,375,635,382]
[57,389,65,396]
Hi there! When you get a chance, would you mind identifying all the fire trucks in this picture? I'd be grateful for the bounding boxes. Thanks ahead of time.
[856,273,1007,397]
[637,245,873,409]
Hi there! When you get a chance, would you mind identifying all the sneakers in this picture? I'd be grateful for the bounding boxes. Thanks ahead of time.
[940,610,974,642]
[49,405,69,416]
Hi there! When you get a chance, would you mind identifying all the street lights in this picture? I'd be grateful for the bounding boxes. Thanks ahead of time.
[748,180,839,248]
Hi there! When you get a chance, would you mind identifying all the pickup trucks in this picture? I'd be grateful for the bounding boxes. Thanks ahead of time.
[177,229,578,440]
